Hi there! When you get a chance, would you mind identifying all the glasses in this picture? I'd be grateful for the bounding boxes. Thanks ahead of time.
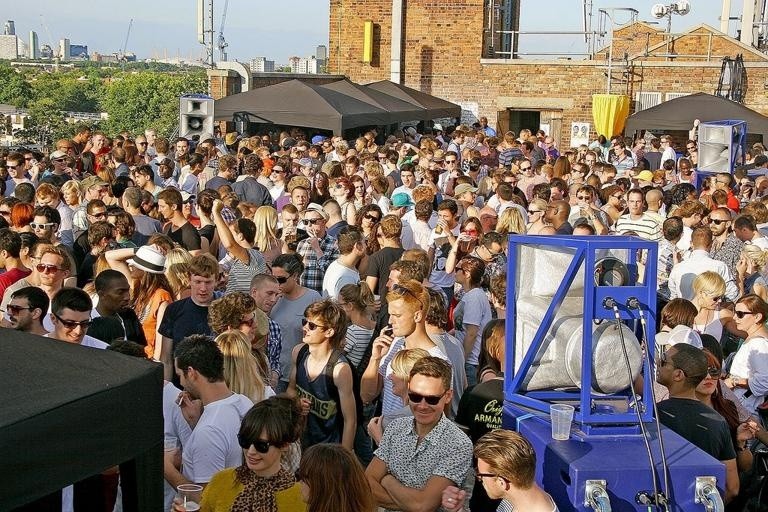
[0,142,755,482]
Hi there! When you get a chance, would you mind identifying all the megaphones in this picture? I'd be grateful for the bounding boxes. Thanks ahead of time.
[506,232,653,439]
[698,121,745,174]
[179,97,214,140]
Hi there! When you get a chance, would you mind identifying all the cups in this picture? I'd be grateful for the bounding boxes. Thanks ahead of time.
[176,482,203,511]
[165,435,175,451]
[461,235,471,251]
[435,223,443,235]
[285,227,297,243]
[579,205,588,217]
[548,402,575,442]
